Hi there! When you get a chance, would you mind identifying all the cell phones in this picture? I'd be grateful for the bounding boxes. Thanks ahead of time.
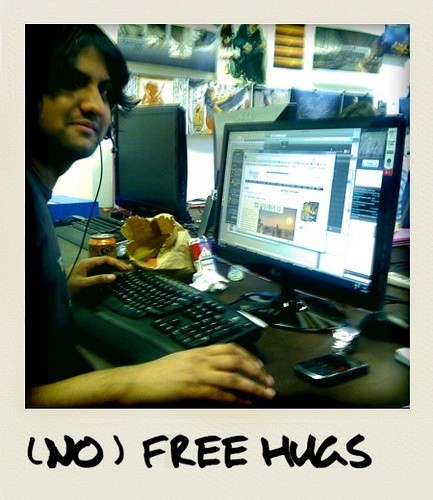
[293,352,369,384]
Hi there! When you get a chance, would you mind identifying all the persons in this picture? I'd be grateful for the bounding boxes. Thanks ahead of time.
[234,25,267,86]
[203,87,228,135]
[24,24,278,408]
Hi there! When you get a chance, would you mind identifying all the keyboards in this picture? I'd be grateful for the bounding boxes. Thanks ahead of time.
[71,266,263,365]
[57,210,128,251]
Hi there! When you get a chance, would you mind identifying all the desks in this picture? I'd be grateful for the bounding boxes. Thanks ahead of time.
[50,206,410,407]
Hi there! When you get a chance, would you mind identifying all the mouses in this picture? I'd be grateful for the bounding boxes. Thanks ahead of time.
[358,311,409,342]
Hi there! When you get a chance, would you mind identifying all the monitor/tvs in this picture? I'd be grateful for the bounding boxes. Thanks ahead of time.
[212,115,406,331]
[114,105,185,218]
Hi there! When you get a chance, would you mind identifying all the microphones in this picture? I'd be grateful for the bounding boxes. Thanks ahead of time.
[107,136,117,153]
